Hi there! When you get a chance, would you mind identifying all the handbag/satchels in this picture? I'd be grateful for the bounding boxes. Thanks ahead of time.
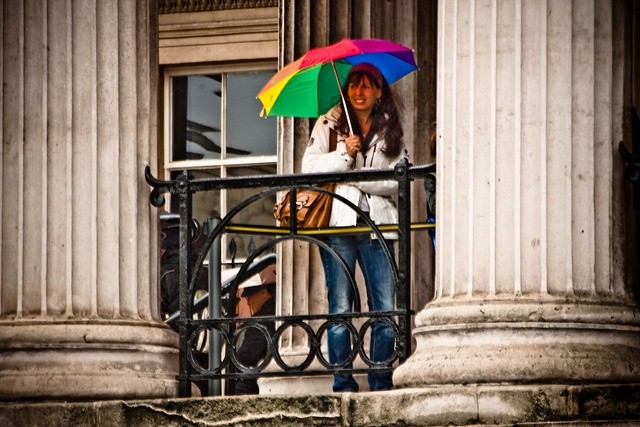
[273,182,335,228]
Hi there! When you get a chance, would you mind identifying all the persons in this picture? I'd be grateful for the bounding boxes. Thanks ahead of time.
[301,61,411,390]
[229,283,276,394]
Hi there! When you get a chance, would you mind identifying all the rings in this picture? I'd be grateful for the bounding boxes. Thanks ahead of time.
[355,142,358,146]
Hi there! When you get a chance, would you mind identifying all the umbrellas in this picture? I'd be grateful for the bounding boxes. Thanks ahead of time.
[255,36,421,137]
[220,263,276,287]
